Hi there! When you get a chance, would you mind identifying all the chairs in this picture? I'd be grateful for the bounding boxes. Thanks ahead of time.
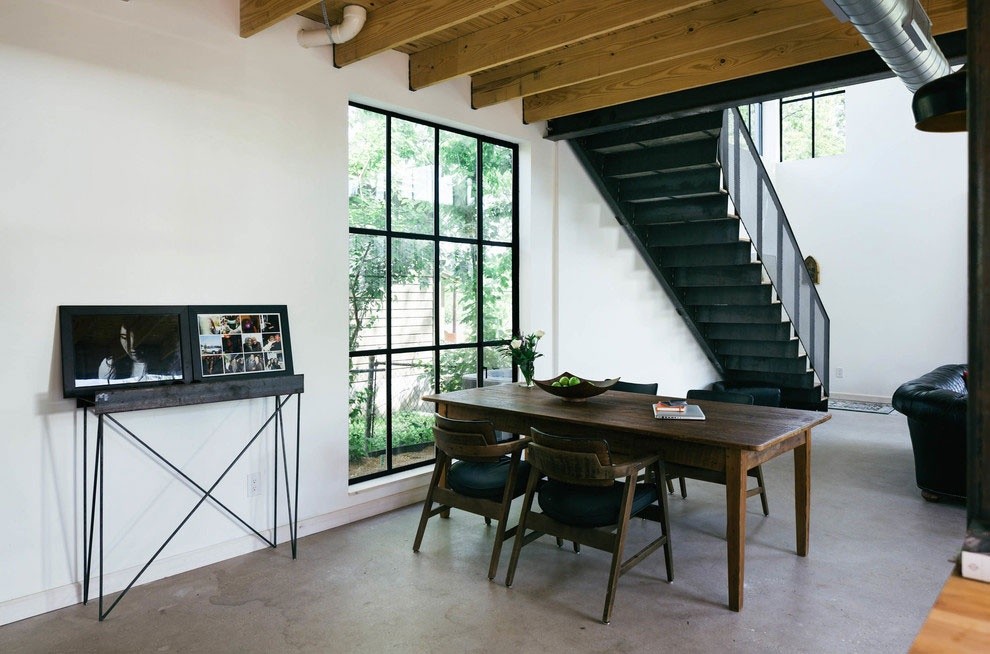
[641,389,771,521]
[504,426,676,627]
[412,411,564,583]
[603,378,676,494]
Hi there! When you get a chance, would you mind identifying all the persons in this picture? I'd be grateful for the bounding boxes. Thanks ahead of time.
[98,316,175,379]
[199,315,283,375]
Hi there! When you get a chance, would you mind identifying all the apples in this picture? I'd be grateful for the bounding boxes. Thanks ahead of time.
[551,376,581,387]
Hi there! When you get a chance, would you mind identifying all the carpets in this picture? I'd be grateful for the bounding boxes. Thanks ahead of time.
[827,397,895,415]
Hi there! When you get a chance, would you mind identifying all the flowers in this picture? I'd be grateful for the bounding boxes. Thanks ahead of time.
[493,328,546,385]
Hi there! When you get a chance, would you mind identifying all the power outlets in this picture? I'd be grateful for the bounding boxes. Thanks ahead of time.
[835,366,845,378]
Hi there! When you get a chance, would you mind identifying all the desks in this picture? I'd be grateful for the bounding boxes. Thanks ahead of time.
[420,379,833,614]
[907,551,990,654]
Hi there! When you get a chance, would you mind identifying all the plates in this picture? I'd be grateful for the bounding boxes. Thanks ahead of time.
[531,372,621,403]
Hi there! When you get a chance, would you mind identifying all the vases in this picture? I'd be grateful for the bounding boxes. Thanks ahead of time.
[516,363,535,387]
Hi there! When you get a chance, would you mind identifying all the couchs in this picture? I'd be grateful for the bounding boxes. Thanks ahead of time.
[891,363,969,504]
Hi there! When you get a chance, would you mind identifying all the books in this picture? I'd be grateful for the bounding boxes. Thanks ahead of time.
[656,400,686,411]
